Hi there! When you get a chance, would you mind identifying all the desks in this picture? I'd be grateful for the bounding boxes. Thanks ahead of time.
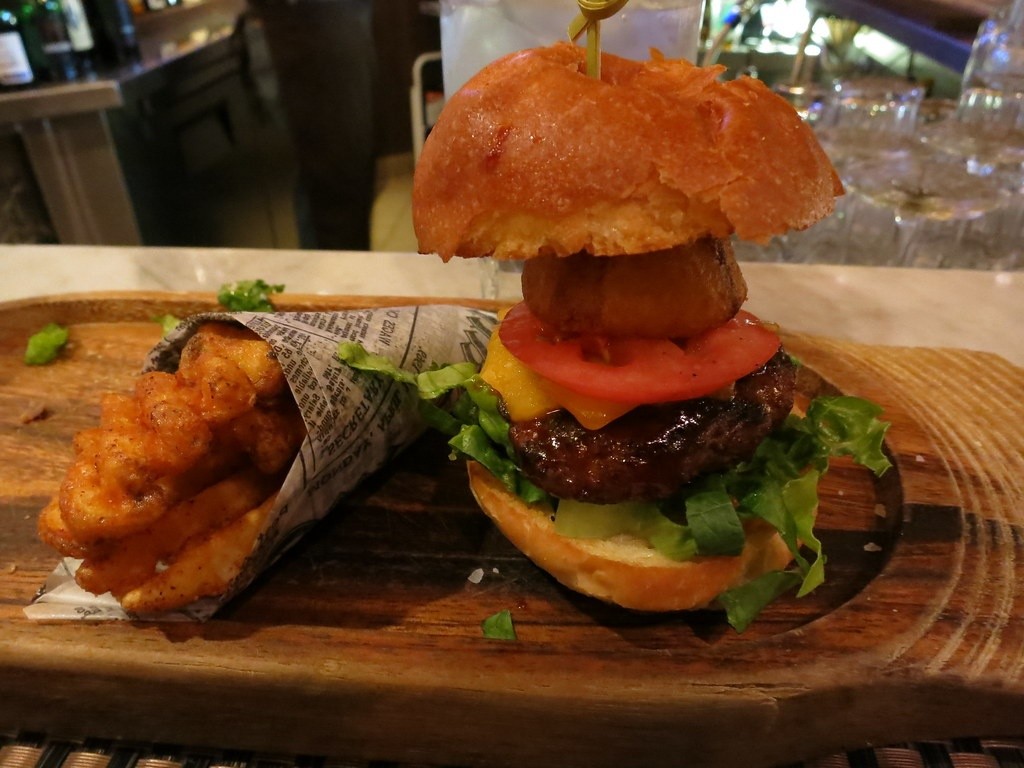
[789,1,1004,90]
[1,243,1024,767]
[0,14,296,243]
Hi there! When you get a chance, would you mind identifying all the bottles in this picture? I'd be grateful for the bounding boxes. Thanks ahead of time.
[0,6,36,91]
[83,0,143,78]
[34,0,101,84]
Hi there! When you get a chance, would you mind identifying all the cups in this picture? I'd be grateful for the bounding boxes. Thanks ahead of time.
[728,0,1024,272]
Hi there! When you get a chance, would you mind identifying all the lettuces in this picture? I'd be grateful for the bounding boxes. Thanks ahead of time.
[22,278,284,365]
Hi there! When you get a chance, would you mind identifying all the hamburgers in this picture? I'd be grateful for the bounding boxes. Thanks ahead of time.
[336,40,892,634]
